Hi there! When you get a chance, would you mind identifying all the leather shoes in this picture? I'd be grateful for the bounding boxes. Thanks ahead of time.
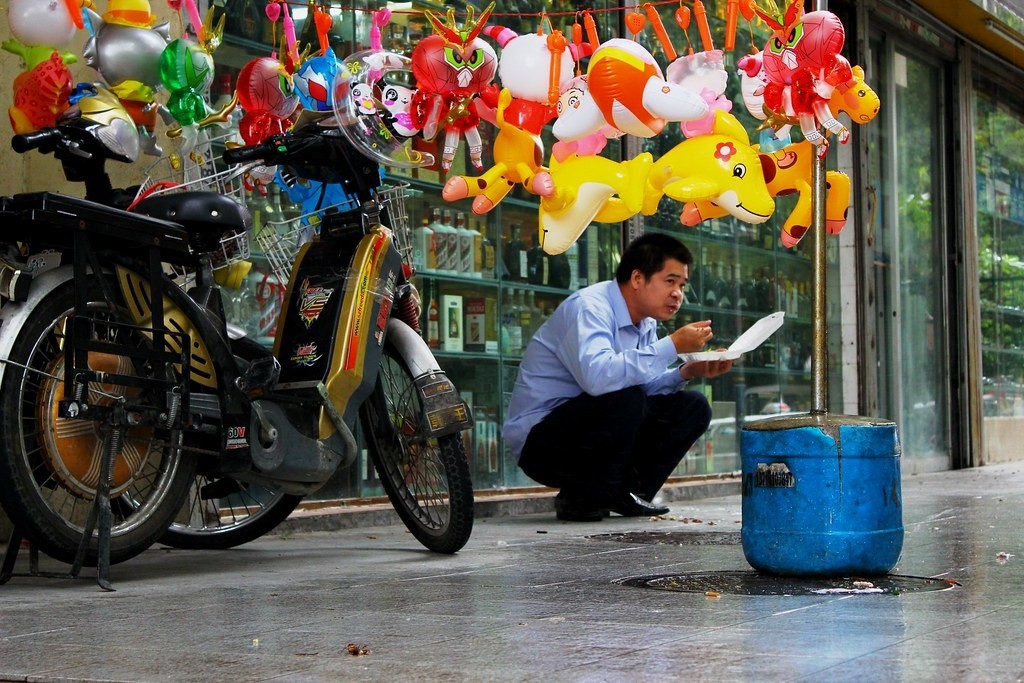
[601,491,669,515]
[556,493,602,521]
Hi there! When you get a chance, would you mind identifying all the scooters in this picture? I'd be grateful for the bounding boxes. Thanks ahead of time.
[0,67,477,592]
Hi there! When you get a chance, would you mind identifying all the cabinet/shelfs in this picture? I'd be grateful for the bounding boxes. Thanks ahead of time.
[352,171,1008,499]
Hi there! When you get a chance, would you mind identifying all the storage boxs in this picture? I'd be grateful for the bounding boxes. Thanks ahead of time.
[463,297,497,352]
[439,294,463,352]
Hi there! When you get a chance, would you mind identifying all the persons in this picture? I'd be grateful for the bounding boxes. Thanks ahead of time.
[504,233,732,521]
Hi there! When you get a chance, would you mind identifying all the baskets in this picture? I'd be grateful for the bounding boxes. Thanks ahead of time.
[131,131,250,287]
[254,178,417,293]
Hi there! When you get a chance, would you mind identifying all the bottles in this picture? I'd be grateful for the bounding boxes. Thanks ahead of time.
[450,312,458,337]
[685,254,811,319]
[429,208,448,275]
[261,0,323,55]
[455,212,472,277]
[213,74,241,143]
[427,280,439,349]
[471,316,480,342]
[505,223,581,290]
[442,209,460,275]
[243,166,304,256]
[464,398,496,472]
[505,288,555,353]
[416,215,436,272]
[480,225,495,278]
[467,218,483,279]
[217,263,288,345]
